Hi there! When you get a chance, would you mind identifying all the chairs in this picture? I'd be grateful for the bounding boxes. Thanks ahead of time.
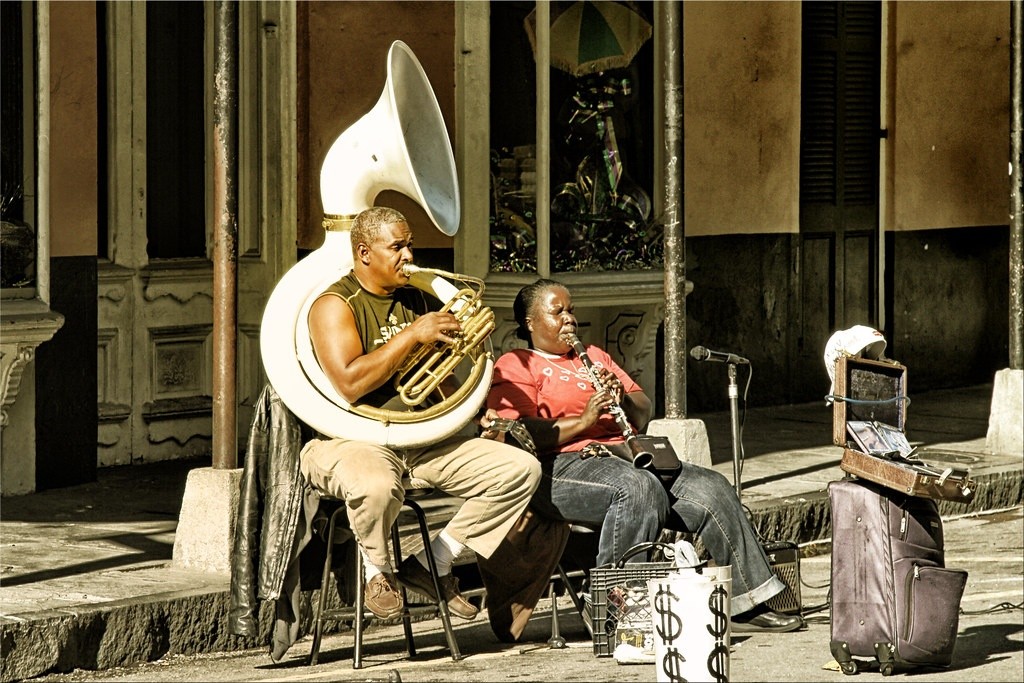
[265,382,593,670]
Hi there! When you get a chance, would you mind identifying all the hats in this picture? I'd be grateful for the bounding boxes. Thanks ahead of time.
[824,325,887,406]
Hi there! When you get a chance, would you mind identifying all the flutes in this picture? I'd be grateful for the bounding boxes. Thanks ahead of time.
[566,331,654,470]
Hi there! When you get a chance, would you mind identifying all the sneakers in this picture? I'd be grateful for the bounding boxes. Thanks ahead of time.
[393,553,478,620]
[363,563,404,621]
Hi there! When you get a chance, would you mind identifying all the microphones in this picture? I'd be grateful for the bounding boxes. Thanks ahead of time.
[689,345,750,364]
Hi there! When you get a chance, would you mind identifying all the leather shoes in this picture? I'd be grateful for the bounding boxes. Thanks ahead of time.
[730,601,803,631]
[579,594,593,629]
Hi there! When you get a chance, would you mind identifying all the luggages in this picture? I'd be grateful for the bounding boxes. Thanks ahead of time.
[826,472,970,675]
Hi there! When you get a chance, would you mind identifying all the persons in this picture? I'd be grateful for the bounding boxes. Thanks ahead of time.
[296,207,542,620]
[489,280,802,632]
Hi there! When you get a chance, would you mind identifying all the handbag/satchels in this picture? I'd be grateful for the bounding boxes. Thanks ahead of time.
[580,435,682,479]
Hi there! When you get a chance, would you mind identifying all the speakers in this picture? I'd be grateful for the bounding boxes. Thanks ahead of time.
[764,541,802,615]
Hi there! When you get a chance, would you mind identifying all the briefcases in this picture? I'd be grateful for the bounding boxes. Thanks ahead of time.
[833,354,978,503]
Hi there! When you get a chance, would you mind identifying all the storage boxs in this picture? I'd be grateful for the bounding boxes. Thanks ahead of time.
[591,561,713,657]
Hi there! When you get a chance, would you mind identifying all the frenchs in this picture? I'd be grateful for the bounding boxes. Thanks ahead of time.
[259,39,497,448]
